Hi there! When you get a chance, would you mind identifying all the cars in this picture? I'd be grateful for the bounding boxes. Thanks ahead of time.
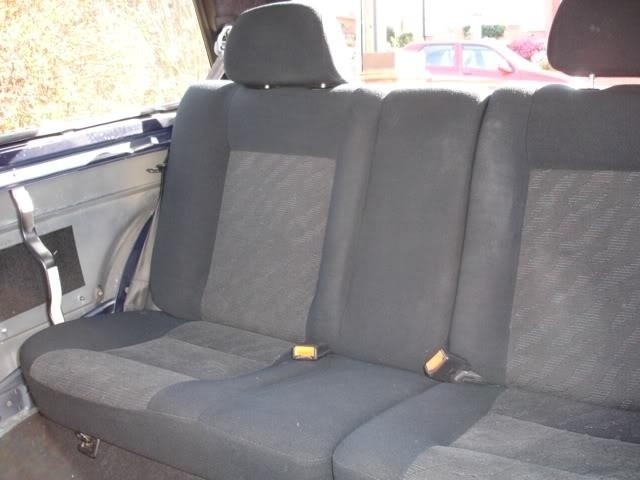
[403,38,566,91]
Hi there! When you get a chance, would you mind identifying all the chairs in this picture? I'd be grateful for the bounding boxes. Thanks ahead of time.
[332,0,640,480]
[18,2,490,480]
